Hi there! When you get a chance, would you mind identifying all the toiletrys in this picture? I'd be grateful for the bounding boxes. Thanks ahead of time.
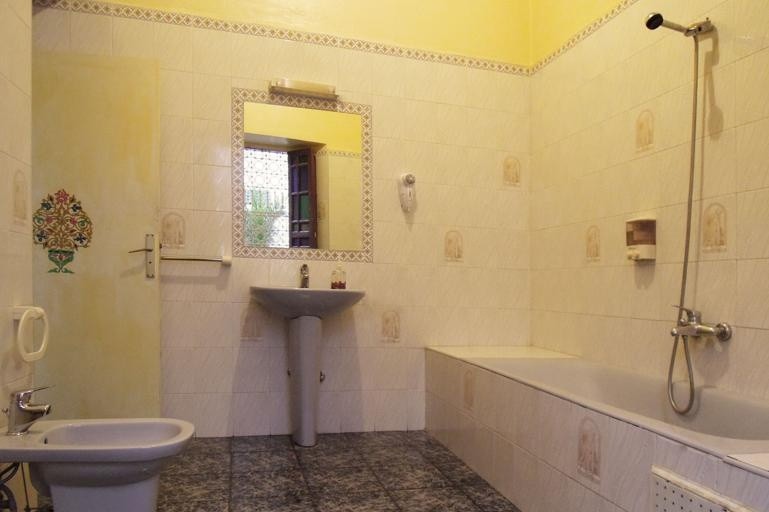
[330,265,348,288]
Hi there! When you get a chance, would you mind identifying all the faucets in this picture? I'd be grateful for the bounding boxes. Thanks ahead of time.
[670,306,702,342]
[300,263,309,286]
[5,386,51,435]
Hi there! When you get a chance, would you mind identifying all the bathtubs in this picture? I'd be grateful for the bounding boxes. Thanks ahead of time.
[425,346,769,510]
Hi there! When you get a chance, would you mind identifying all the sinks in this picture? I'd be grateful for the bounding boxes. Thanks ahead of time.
[0,419,196,512]
[250,285,366,318]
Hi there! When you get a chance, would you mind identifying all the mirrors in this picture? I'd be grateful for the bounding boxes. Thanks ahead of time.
[231,88,373,264]
